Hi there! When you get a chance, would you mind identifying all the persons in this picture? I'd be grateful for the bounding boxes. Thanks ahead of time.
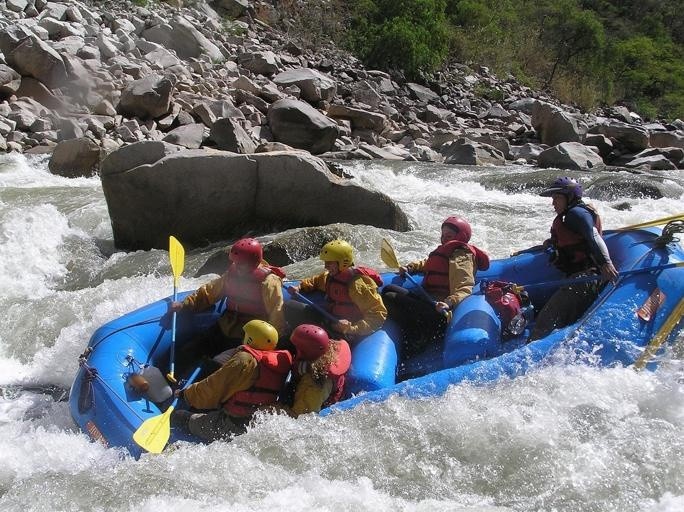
[258,323,352,418]
[170,319,293,442]
[284,240,388,350]
[169,238,287,380]
[526,176,619,346]
[380,216,489,341]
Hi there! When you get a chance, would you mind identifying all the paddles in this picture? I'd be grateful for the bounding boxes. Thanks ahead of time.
[602,212,684,237]
[166,236,185,383]
[132,367,199,454]
[381,238,452,323]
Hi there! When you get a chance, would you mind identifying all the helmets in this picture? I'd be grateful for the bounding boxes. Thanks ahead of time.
[242,320,278,351]
[231,238,263,272]
[290,324,328,359]
[540,176,582,198]
[320,240,353,272]
[442,216,471,243]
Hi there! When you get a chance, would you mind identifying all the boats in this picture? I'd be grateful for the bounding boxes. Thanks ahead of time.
[66,224,683,460]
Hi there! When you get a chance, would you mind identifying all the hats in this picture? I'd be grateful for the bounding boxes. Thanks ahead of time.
[539,188,560,196]
